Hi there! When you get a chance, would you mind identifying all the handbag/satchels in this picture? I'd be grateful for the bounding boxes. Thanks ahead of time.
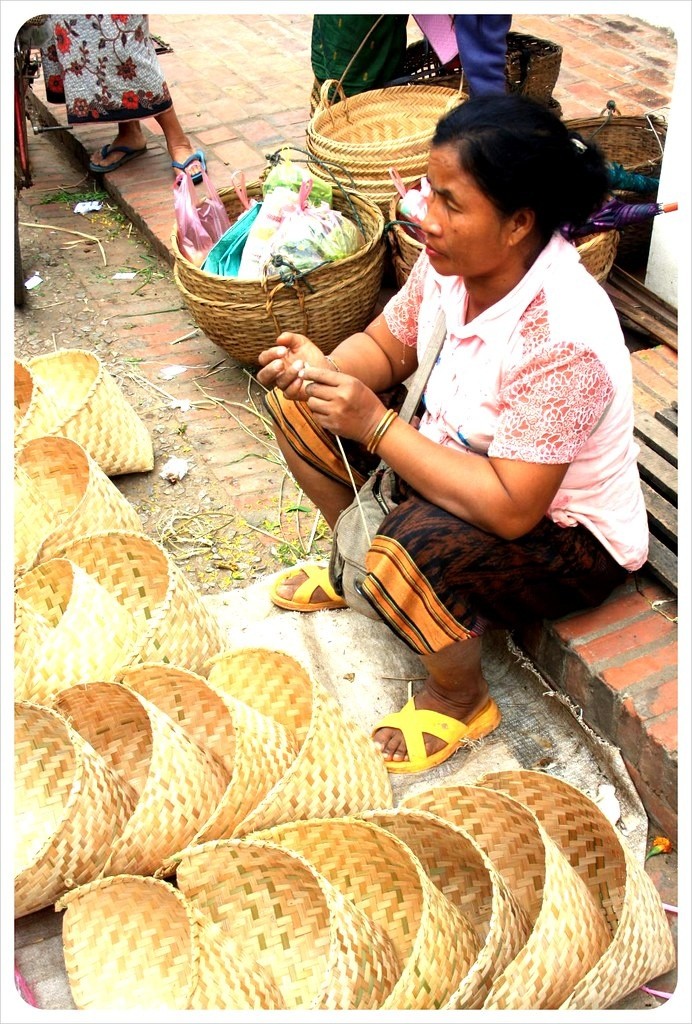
[173,147,364,280]
[330,459,400,623]
[389,166,431,247]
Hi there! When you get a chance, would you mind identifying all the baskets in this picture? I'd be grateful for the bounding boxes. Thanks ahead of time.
[173,187,386,364]
[308,80,468,209]
[385,177,621,295]
[387,30,564,119]
[561,117,668,248]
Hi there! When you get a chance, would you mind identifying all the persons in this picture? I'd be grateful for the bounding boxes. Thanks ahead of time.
[453,14,512,99]
[39,15,206,183]
[257,98,650,774]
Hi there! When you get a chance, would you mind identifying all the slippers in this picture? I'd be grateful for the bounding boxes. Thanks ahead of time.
[270,565,350,612]
[370,695,501,773]
[89,143,147,172]
[172,150,207,186]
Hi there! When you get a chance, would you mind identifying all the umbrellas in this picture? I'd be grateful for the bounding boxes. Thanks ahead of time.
[558,162,679,241]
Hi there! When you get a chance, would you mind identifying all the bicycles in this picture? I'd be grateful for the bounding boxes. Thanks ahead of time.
[14,33,73,308]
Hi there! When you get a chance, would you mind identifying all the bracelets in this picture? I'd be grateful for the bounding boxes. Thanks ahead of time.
[367,409,393,451]
[325,355,341,372]
[371,412,398,454]
